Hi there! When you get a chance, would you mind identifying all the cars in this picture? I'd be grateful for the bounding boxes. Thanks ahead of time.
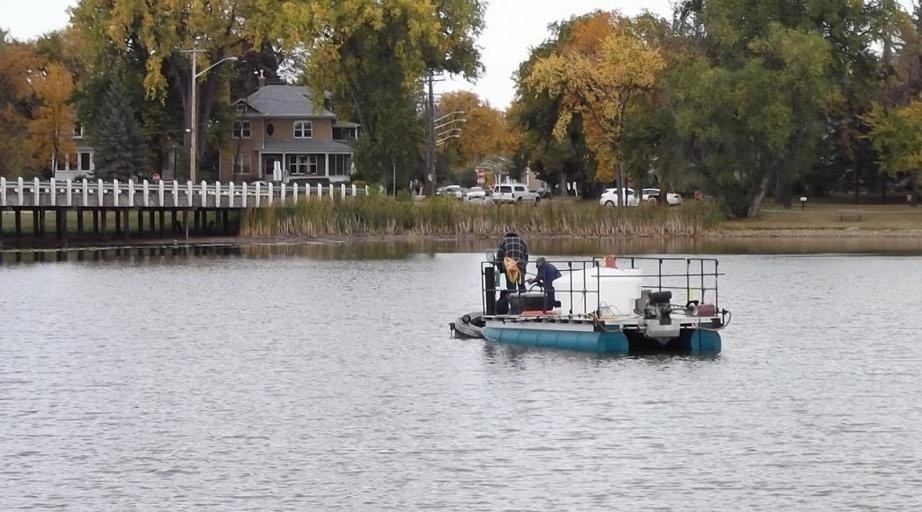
[535,188,551,199]
[600,188,654,207]
[434,183,487,201]
[639,188,682,206]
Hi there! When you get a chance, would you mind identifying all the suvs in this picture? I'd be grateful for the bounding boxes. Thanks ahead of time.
[491,183,542,207]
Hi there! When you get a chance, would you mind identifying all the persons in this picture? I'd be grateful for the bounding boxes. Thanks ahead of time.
[527,257,562,294]
[494,232,528,292]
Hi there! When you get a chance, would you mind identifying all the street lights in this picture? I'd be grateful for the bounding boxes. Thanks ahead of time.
[472,154,511,207]
[187,46,241,195]
[425,109,467,196]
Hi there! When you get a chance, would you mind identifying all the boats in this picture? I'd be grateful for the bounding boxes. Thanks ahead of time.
[475,243,733,359]
